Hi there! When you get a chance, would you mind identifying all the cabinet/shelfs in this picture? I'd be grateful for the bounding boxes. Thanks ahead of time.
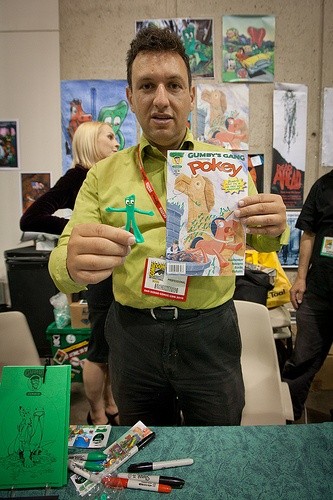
[4,244,89,357]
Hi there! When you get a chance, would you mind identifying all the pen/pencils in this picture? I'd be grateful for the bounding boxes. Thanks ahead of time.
[86,432,155,480]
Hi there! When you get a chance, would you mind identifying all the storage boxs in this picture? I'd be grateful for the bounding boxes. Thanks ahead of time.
[70,301,95,329]
[46,321,93,380]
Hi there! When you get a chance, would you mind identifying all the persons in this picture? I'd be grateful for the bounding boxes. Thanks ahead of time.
[48,26,290,425]
[19,121,121,428]
[283,167,333,425]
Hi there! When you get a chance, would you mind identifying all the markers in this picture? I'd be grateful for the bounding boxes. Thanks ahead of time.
[127,458,193,473]
[117,473,185,489]
[68,451,108,461]
[68,461,101,484]
[68,459,105,472]
[101,477,171,493]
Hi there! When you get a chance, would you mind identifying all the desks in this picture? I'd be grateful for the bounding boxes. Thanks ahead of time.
[0,425,333,500]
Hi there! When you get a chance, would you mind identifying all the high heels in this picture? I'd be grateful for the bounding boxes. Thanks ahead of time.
[87,408,121,426]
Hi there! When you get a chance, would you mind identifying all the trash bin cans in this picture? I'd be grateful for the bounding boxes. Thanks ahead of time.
[46,321,94,383]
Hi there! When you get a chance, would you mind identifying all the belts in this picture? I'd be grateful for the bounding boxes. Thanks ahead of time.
[132,305,213,321]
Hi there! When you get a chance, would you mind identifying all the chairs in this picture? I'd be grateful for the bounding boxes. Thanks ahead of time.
[0,310,41,371]
[232,299,297,425]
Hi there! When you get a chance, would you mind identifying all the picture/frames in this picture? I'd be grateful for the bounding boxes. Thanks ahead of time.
[276,211,305,268]
[0,118,21,170]
[18,170,53,216]
